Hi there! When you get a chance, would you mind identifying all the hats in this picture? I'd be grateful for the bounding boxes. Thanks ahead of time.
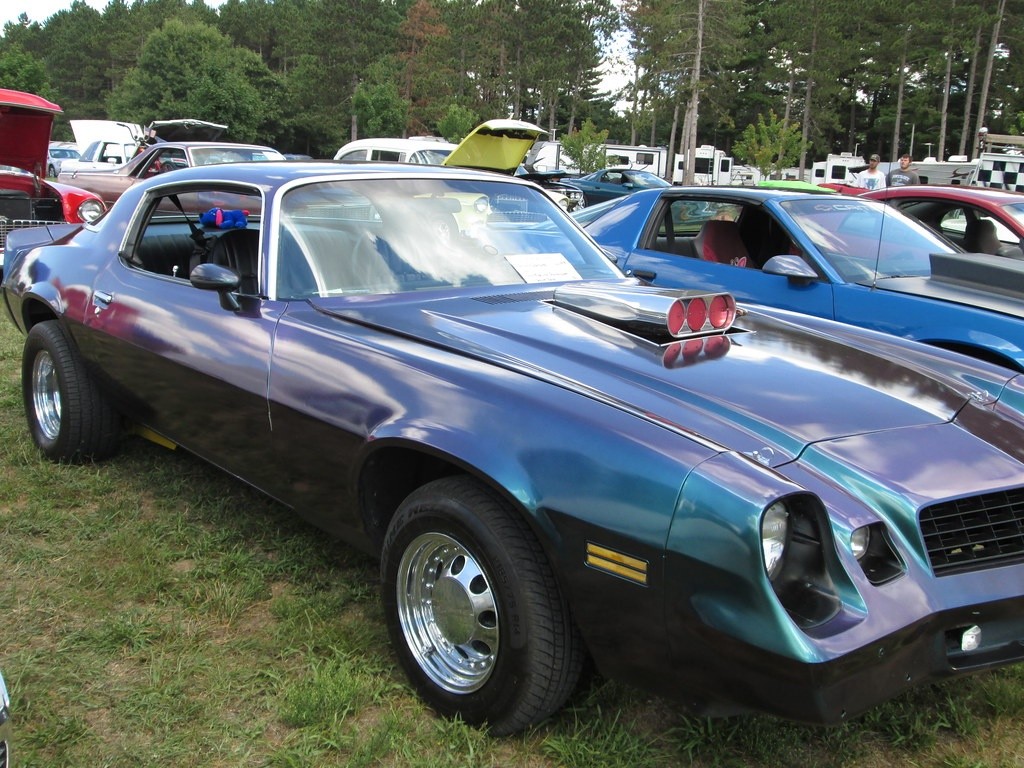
[870,154,880,162]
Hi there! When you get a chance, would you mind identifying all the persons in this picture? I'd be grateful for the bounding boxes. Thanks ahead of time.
[886,155,918,188]
[965,218,1003,256]
[856,154,886,190]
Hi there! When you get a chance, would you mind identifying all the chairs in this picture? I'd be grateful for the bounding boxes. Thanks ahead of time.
[209,229,259,295]
[692,219,754,269]
[963,221,1002,254]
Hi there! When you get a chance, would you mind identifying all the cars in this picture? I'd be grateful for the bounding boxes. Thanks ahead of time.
[0,157,1023,739]
[0,114,1024,383]
